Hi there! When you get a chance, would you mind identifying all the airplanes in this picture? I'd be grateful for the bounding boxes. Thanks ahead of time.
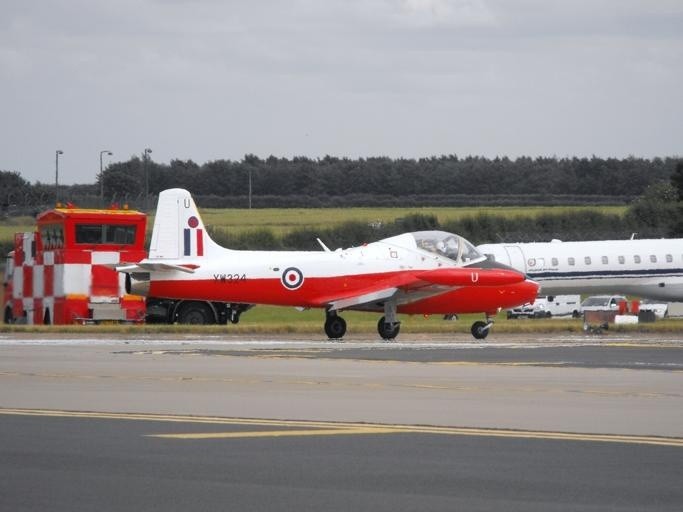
[114,188,540,339]
[476,233,683,303]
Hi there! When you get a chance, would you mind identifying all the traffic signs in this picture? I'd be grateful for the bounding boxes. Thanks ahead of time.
[144,147,152,209]
[248,167,253,208]
[100,150,112,208]
[55,150,62,200]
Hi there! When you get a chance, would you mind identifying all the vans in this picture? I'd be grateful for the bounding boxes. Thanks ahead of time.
[506,294,580,319]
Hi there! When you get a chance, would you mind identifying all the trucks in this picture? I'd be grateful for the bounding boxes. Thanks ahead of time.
[2,202,148,324]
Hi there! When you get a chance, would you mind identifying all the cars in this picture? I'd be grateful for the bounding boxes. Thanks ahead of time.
[637,298,667,318]
[572,295,629,319]
